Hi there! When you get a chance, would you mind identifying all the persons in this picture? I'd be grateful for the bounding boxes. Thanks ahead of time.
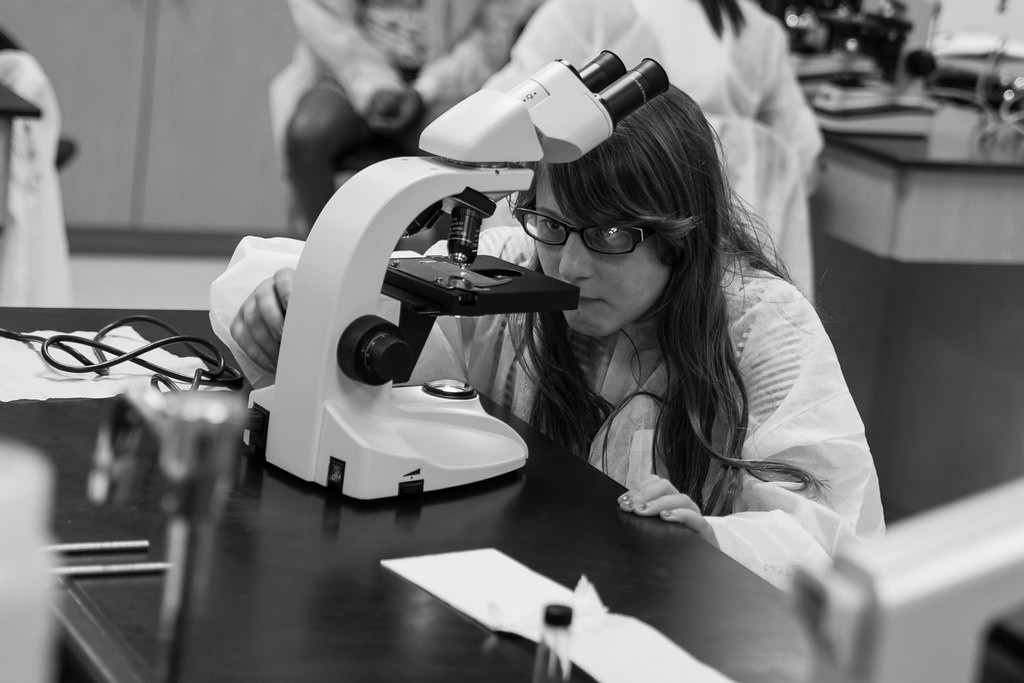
[0,30,75,307]
[479,0,826,308]
[211,81,887,593]
[269,0,543,225]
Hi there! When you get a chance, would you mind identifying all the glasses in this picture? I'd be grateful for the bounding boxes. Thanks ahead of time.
[516,195,656,254]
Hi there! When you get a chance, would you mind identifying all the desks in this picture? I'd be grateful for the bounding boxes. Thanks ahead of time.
[0,307,876,683]
[807,104,1024,524]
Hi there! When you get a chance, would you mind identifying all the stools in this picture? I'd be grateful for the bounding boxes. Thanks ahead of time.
[289,135,410,237]
